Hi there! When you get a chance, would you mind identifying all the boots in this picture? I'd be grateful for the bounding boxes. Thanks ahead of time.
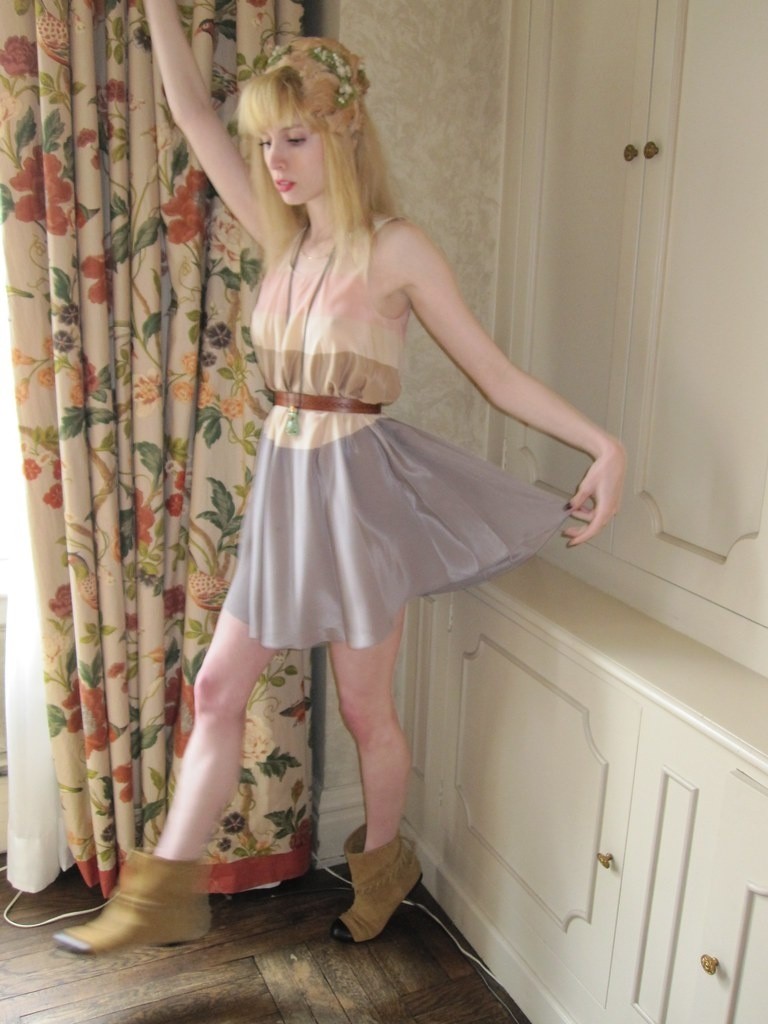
[330,823,423,944]
[52,847,212,954]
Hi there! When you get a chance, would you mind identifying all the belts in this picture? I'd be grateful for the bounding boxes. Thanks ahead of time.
[273,391,382,414]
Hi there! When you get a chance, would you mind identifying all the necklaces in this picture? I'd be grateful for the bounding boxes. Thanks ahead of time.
[280,221,338,434]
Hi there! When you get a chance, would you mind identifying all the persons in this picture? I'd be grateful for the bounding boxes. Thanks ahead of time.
[54,1,627,957]
